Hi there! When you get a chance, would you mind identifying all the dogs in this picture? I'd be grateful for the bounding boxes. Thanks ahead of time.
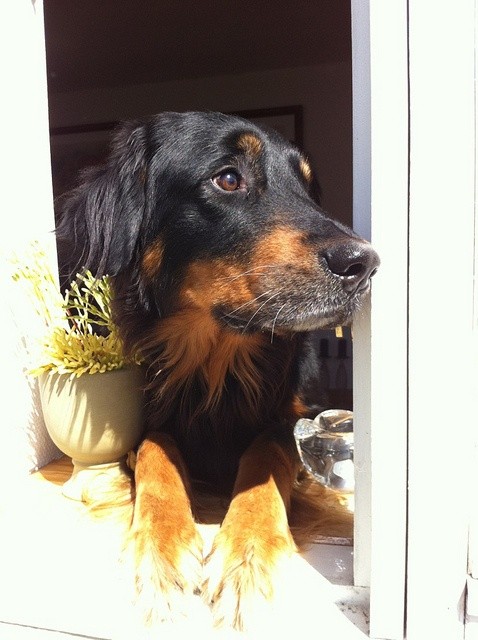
[52,107,383,637]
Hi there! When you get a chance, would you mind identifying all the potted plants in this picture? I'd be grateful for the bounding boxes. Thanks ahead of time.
[13,243,142,511]
